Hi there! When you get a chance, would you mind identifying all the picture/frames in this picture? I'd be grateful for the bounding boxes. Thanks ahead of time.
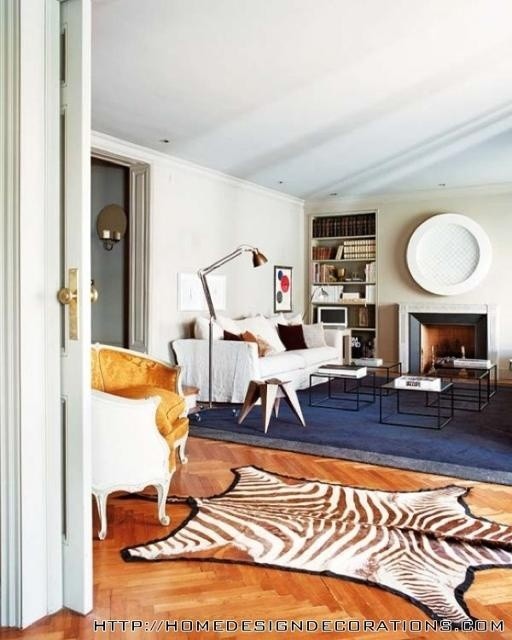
[273,264,293,313]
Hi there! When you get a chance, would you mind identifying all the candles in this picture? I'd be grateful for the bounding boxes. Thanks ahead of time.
[102,229,111,238]
[113,232,121,241]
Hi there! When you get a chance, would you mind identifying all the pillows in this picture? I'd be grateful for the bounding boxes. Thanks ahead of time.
[190,305,330,358]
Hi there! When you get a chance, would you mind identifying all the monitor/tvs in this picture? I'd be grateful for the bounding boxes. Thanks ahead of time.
[317,306,348,329]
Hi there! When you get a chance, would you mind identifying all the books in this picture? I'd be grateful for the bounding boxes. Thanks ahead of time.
[318,363,367,379]
[311,213,377,328]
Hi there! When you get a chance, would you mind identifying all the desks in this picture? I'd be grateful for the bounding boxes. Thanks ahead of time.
[308,357,498,432]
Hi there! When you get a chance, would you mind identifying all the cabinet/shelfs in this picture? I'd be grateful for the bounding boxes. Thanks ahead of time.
[307,208,380,359]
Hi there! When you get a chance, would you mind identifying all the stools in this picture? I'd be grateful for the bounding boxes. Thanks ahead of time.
[182,385,200,409]
[235,375,307,436]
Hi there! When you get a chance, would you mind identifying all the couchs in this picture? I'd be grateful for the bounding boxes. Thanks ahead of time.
[168,322,345,409]
[82,340,193,543]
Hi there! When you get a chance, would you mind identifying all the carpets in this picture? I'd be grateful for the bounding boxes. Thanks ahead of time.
[179,370,511,487]
[110,457,512,633]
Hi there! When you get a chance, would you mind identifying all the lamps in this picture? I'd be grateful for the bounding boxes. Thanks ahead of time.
[194,243,268,321]
